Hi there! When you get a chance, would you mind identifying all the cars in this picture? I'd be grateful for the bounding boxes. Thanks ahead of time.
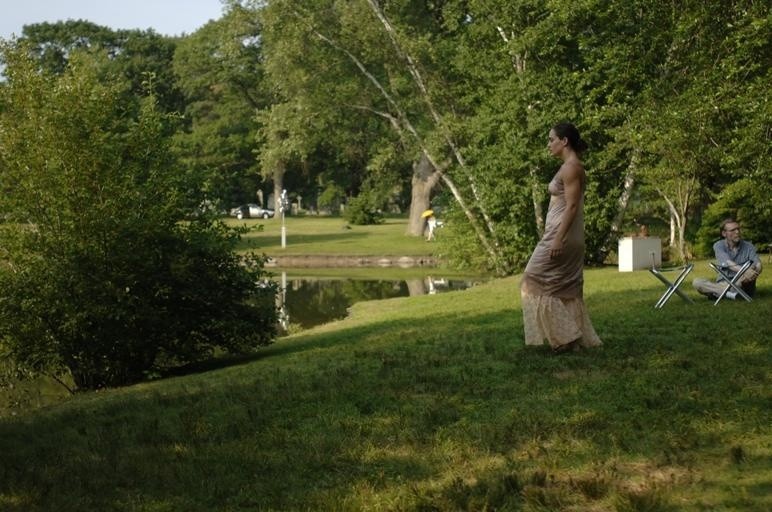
[230,203,275,220]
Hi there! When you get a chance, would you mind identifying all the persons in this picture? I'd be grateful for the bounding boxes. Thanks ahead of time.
[692,218,762,300]
[631,224,650,239]
[427,276,437,295]
[426,215,437,242]
[519,123,607,352]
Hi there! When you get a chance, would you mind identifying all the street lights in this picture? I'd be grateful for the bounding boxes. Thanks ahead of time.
[277,189,289,248]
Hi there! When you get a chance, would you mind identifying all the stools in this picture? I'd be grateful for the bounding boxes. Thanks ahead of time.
[649,259,694,310]
[709,261,758,304]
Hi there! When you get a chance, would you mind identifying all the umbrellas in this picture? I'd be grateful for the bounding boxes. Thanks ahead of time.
[421,210,434,218]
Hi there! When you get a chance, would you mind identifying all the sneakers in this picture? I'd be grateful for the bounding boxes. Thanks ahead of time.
[708,291,740,300]
